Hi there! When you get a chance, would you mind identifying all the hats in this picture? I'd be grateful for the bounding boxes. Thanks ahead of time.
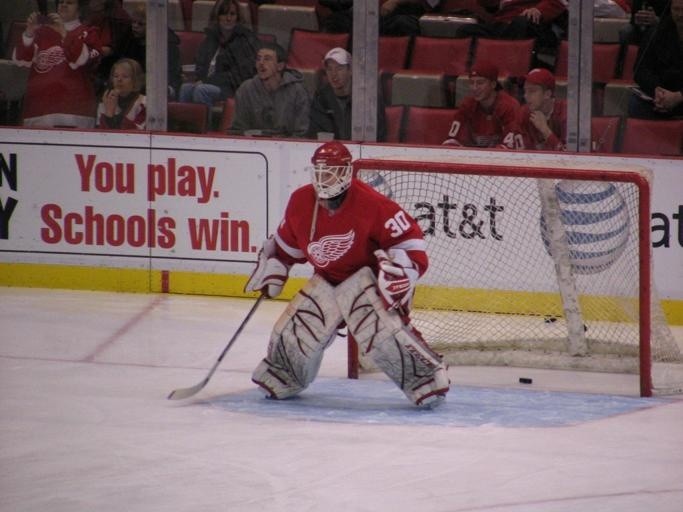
[523,69,555,90]
[324,47,352,65]
[470,62,504,91]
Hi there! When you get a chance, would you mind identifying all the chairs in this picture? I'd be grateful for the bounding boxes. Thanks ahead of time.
[0,0,683,156]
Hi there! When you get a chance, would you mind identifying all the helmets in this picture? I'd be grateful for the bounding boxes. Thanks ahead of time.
[312,142,353,200]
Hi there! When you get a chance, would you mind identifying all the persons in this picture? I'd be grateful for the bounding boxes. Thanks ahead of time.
[316,1,569,75]
[442,61,608,155]
[619,1,683,120]
[227,41,386,141]
[178,2,257,133]
[238,136,450,410]
[13,2,182,132]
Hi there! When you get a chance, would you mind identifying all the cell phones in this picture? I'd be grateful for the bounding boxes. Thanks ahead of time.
[103,81,112,95]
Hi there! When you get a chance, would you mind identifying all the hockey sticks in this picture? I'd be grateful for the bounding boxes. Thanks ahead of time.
[168,293,263,399]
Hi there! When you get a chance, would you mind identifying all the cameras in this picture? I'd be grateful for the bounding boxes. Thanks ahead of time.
[36,15,54,24]
